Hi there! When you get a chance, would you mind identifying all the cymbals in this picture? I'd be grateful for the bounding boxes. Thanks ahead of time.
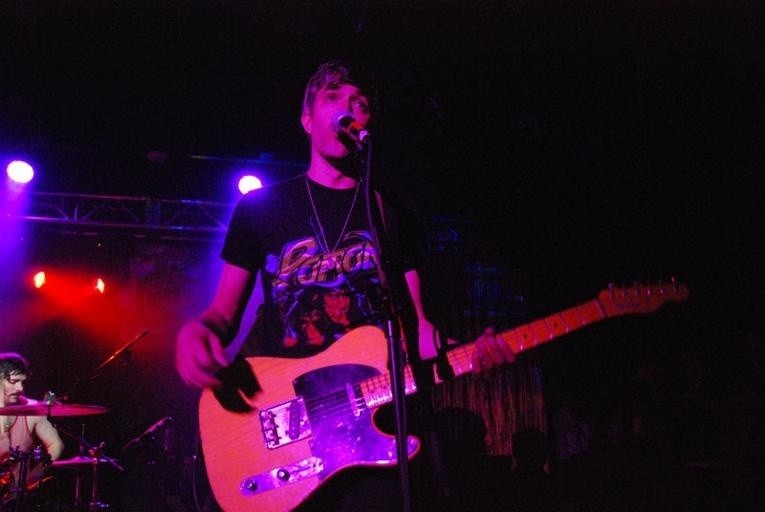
[0,401,108,416]
[48,456,116,466]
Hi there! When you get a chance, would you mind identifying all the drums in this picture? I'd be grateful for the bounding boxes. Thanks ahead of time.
[0,475,73,511]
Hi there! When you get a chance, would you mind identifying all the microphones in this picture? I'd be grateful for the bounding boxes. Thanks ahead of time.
[334,114,371,143]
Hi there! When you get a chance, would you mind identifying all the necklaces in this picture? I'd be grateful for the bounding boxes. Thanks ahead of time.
[300,168,361,272]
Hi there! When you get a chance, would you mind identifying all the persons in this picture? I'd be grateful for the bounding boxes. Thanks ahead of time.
[411,400,518,512]
[505,423,568,512]
[0,350,65,481]
[171,56,519,511]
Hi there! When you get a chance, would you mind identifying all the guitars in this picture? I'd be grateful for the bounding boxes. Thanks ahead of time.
[198,277,688,511]
